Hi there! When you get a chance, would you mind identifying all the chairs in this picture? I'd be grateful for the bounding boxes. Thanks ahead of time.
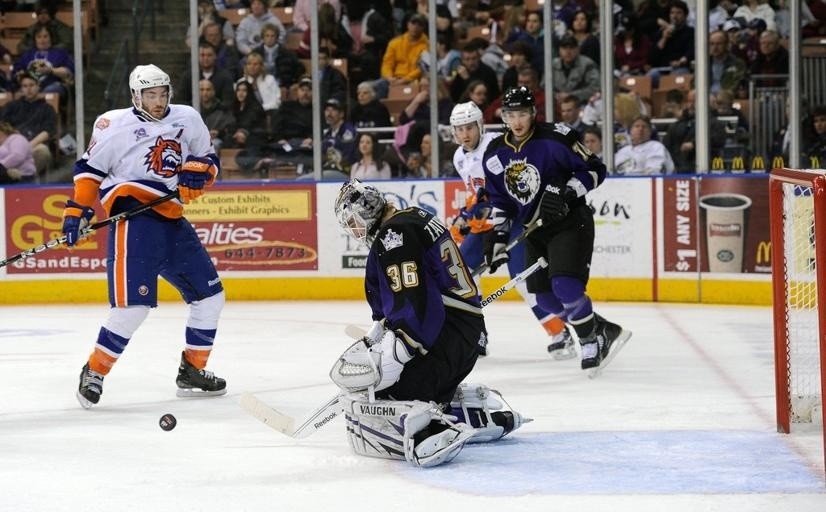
[0,0,101,164]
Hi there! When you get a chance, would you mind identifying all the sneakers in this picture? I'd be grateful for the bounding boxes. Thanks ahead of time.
[546,327,575,356]
[411,419,477,466]
[79,362,104,405]
[175,350,226,393]
[595,315,622,361]
[457,405,532,442]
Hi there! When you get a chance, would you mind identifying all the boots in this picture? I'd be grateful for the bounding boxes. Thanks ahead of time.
[580,335,600,372]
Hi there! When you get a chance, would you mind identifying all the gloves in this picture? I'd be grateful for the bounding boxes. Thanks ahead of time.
[451,208,468,241]
[540,177,567,225]
[177,154,219,203]
[342,330,417,393]
[63,200,95,246]
[486,230,510,273]
[328,321,387,390]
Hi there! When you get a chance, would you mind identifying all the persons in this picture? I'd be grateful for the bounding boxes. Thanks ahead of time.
[325,178,530,470]
[446,99,578,354]
[181,1,825,172]
[481,85,625,372]
[59,63,233,405]
[0,1,74,178]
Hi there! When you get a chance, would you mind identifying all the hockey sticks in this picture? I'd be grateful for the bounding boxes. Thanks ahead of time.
[235,258,549,440]
[345,205,573,341]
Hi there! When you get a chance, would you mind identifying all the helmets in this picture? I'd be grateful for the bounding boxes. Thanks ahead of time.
[449,101,487,150]
[333,178,387,242]
[500,85,536,123]
[128,64,173,125]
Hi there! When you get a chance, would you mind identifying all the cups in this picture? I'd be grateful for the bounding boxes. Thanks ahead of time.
[695,192,753,274]
